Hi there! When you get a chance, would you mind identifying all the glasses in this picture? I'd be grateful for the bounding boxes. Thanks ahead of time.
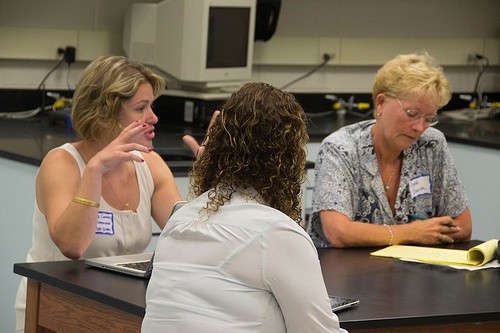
[386,94,439,127]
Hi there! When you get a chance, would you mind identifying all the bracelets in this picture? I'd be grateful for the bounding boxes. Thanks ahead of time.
[384,224,393,245]
[71,196,100,208]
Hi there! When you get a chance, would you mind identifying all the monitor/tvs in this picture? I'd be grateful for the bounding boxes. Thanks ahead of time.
[122,0,257,91]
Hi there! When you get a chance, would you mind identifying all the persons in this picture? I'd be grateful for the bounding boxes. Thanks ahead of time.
[142,82,348,333]
[14,56,220,333]
[306,54,472,249]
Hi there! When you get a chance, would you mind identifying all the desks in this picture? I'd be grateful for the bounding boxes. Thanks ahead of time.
[0,113,500,333]
[14,239,500,333]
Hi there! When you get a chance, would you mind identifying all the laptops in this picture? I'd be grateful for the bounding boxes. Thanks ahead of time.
[85,202,189,278]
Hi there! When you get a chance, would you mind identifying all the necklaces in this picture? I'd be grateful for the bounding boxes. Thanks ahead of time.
[386,170,394,190]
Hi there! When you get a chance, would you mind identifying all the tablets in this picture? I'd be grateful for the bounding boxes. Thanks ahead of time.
[328,295,361,311]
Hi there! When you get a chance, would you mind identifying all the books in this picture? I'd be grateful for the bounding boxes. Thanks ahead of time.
[370,239,498,267]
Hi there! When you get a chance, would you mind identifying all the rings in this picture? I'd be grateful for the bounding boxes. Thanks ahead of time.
[441,235,445,242]
